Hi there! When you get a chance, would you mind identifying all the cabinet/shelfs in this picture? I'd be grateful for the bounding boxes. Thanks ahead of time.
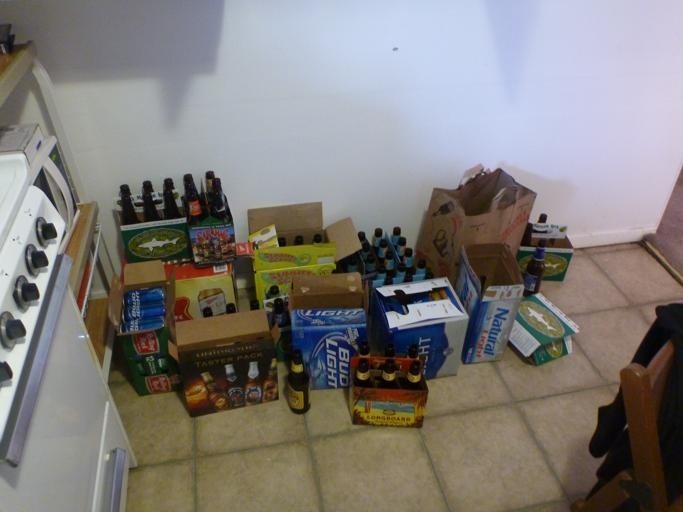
[1,40,122,379]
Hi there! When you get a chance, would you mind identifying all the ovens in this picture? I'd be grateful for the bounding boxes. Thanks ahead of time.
[3,255,141,510]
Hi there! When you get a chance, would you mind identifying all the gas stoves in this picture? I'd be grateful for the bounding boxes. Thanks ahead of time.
[0,148,66,444]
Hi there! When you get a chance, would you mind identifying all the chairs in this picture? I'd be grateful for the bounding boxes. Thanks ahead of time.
[617,340,681,511]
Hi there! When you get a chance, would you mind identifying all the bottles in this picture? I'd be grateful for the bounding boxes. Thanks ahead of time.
[355,224,425,284]
[354,340,425,386]
[199,296,261,318]
[200,359,279,412]
[524,236,546,296]
[272,231,323,246]
[268,283,288,325]
[116,168,226,226]
[285,351,312,417]
[430,201,456,219]
[478,272,486,289]
[533,209,548,233]
